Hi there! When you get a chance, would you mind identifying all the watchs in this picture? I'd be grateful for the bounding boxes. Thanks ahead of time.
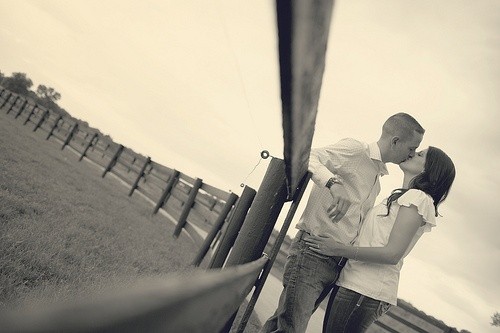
[326,176,342,189]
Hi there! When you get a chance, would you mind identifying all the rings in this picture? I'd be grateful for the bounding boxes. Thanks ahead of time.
[317,244,319,249]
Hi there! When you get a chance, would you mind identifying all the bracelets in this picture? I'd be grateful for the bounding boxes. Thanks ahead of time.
[355,246,359,261]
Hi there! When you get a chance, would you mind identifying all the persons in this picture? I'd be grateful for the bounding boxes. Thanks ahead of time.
[260,112,426,332]
[304,145,457,333]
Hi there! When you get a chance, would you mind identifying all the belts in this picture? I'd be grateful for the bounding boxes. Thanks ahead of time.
[301,232,348,266]
